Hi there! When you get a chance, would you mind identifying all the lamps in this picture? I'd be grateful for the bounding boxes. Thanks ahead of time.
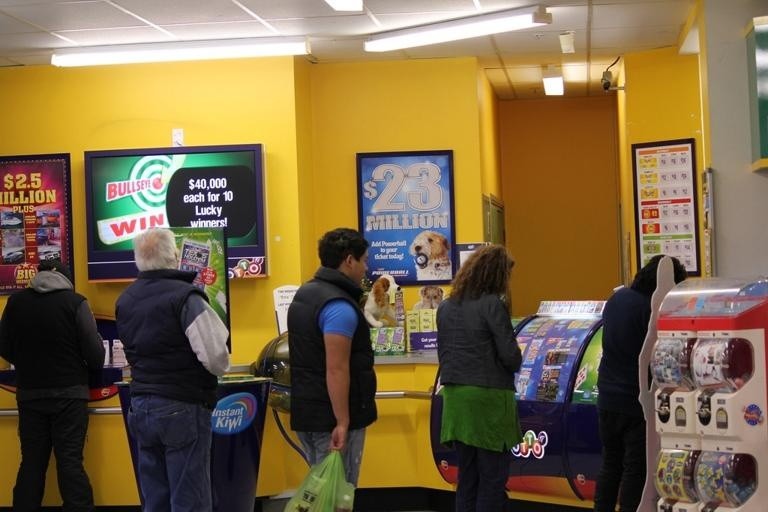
[362,4,552,53]
[50,35,312,67]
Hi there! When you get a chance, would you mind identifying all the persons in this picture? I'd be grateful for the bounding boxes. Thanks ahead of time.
[287,228,377,488]
[115,227,229,512]
[436,243,523,512]
[0,259,106,512]
[594,255,689,512]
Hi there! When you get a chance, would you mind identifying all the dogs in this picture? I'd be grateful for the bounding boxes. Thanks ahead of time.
[413,285,445,311]
[410,230,452,281]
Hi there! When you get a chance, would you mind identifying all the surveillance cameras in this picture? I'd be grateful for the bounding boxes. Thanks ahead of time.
[602,71,612,92]
